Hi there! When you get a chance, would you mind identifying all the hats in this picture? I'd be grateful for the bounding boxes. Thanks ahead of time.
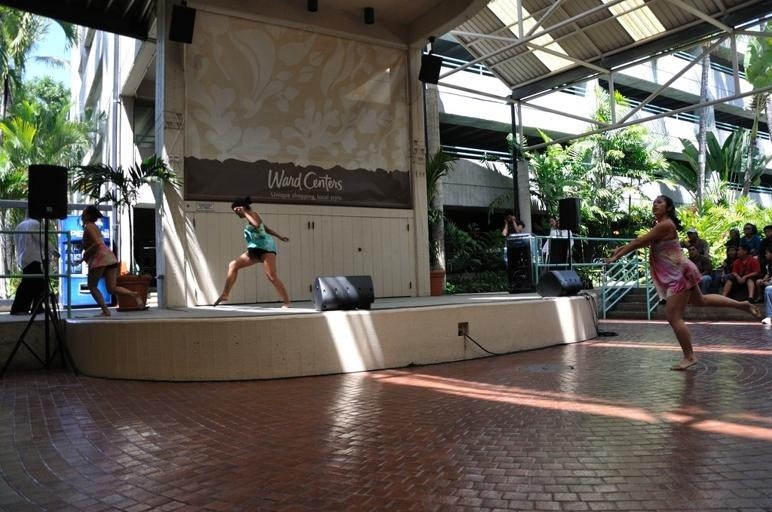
[688,228,695,234]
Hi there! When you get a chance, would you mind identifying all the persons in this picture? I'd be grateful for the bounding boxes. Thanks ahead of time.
[10,217,60,316]
[542,216,574,258]
[501,209,525,272]
[214,196,293,311]
[606,195,762,370]
[681,223,772,324]
[72,205,144,317]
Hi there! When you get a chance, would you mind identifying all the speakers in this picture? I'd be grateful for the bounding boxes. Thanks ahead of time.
[419,54,443,84]
[558,198,582,230]
[28,165,68,220]
[549,239,577,271]
[312,275,374,311]
[507,233,539,294]
[536,270,583,297]
[169,5,196,44]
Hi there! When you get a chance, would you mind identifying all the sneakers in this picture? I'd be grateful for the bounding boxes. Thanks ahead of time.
[762,317,771,324]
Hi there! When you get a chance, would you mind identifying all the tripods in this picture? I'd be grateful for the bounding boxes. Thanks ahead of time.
[0,219,79,378]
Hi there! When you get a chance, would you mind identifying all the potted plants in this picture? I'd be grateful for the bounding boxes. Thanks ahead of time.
[426,146,460,295]
[67,147,186,311]
